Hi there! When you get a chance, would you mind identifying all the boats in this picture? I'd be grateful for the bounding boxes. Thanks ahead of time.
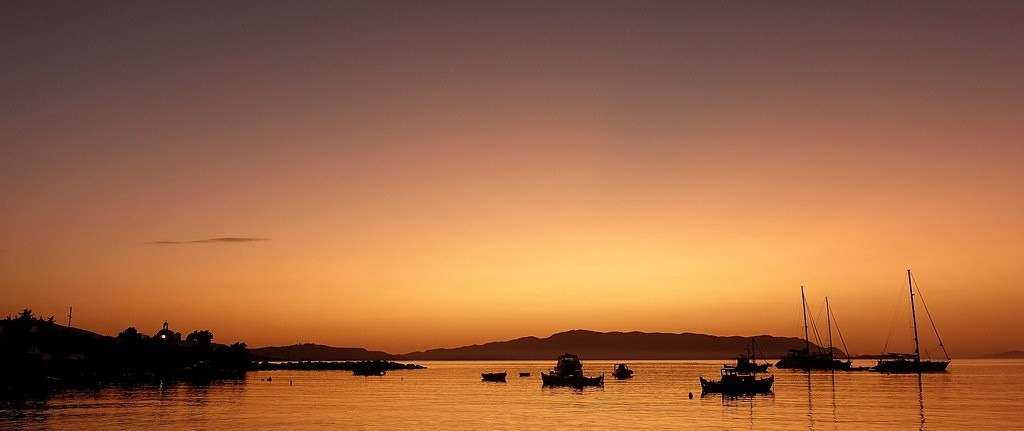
[352,367,385,376]
[700,368,774,394]
[482,371,507,382]
[541,353,604,390]
[723,337,774,375]
[612,364,633,379]
[519,372,530,376]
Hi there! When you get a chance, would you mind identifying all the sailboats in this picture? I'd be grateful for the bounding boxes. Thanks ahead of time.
[774,285,870,370]
[868,270,951,373]
